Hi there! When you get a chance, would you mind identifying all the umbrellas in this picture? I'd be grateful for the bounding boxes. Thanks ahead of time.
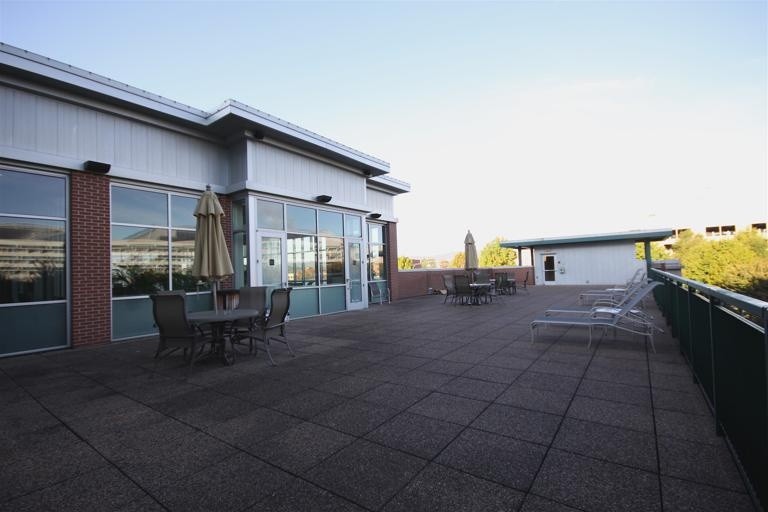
[463,229,480,283]
[192,183,236,312]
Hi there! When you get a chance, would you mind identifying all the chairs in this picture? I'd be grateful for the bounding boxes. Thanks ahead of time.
[441,270,529,308]
[545,278,664,334]
[529,280,665,354]
[579,273,648,309]
[606,268,642,295]
[149,286,296,368]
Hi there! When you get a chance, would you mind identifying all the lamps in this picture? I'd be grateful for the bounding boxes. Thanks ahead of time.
[370,214,382,218]
[316,195,333,203]
[84,160,111,174]
